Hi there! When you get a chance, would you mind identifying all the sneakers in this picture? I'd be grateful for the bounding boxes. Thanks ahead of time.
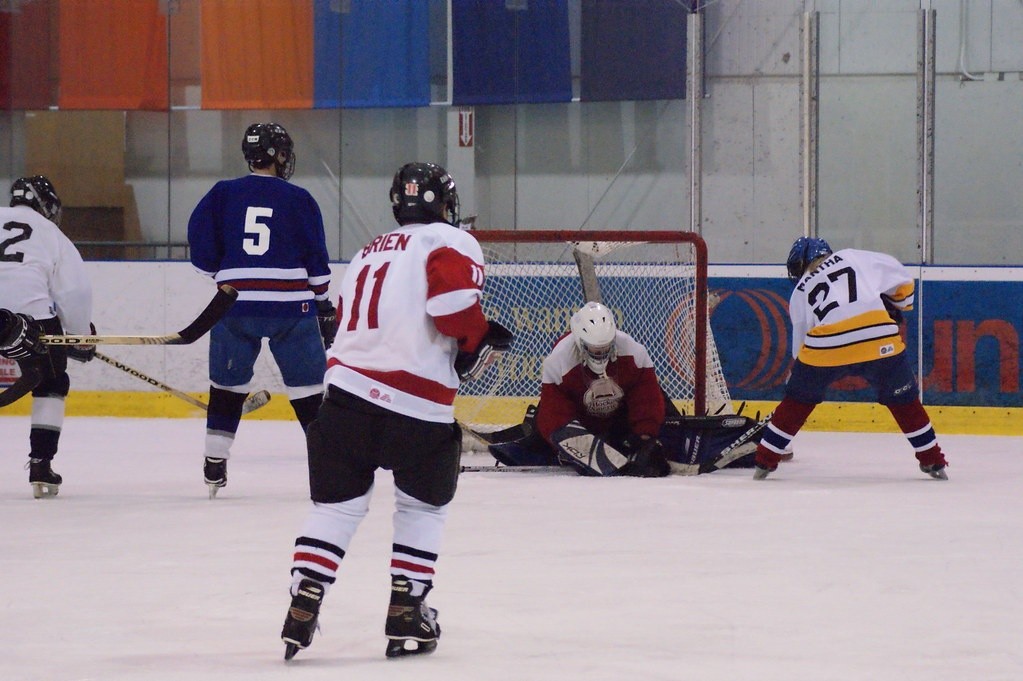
[385,574,440,657]
[281,579,324,660]
[24,456,62,498]
[919,462,949,480]
[203,456,226,499]
[753,462,778,479]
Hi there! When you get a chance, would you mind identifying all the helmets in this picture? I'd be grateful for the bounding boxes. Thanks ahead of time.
[10,175,62,227]
[390,161,460,228]
[242,122,296,180]
[787,236,832,284]
[569,301,616,374]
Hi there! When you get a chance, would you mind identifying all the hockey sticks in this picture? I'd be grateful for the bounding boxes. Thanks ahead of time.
[37,284,239,345]
[93,348,271,416]
[457,442,758,475]
[668,409,775,476]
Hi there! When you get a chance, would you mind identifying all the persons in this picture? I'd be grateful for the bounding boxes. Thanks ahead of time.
[754,237,948,480]
[456,299,769,477]
[275,161,513,663]
[0,174,99,500]
[185,119,333,497]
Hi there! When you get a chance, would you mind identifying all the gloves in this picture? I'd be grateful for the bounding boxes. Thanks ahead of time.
[66,321,97,363]
[455,321,514,383]
[317,304,337,350]
